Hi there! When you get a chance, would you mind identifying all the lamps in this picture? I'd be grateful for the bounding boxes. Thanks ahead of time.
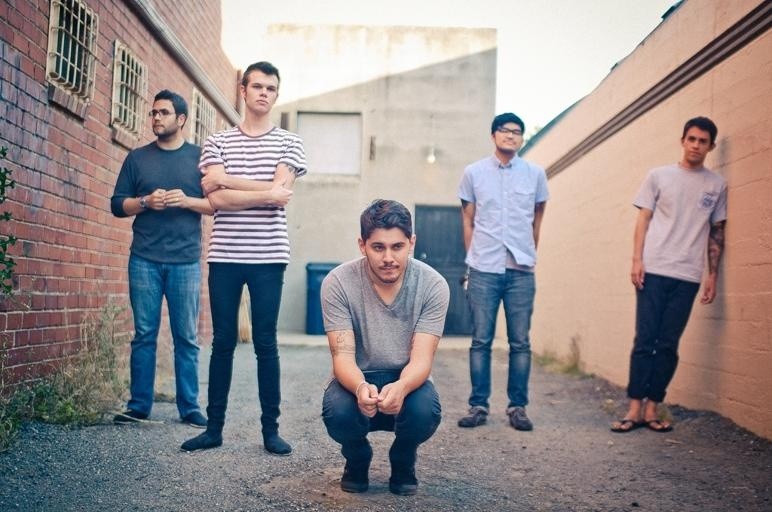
[424,95,441,167]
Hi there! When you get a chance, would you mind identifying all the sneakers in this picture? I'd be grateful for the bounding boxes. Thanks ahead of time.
[389,439,417,496]
[341,439,373,493]
[264,436,293,456]
[180,431,222,451]
[509,408,532,431]
[182,412,207,428]
[113,411,147,425]
[458,408,486,427]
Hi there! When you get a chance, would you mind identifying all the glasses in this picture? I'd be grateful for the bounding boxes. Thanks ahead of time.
[149,111,176,116]
[499,128,521,134]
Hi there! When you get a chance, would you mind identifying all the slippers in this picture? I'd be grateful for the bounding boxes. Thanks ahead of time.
[611,420,673,432]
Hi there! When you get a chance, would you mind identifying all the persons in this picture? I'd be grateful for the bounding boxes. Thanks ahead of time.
[177,63,309,456]
[109,90,216,427]
[458,113,547,429]
[612,118,728,433]
[316,199,451,496]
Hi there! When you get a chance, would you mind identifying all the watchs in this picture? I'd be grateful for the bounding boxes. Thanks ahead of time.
[139,196,147,209]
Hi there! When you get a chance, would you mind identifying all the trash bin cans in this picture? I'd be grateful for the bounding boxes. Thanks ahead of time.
[306,262,338,335]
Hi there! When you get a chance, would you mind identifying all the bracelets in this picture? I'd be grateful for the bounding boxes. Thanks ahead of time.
[354,380,369,399]
